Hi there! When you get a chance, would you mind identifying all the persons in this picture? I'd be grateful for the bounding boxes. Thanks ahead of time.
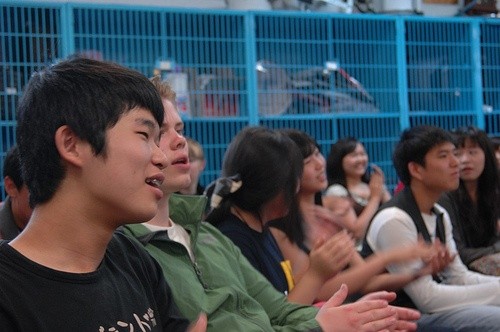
[178,124,500,332]
[119,77,420,332]
[0,56,207,332]
[0,147,34,241]
